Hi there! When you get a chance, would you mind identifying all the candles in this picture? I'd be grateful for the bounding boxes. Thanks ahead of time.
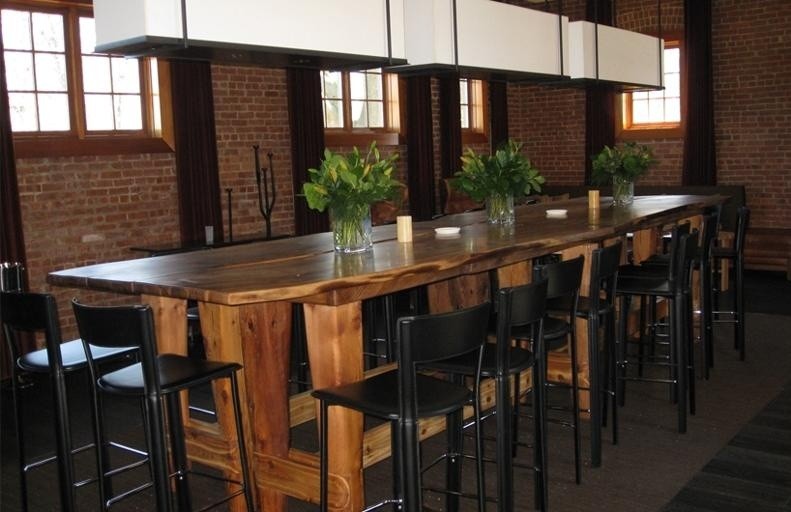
[205,224,214,246]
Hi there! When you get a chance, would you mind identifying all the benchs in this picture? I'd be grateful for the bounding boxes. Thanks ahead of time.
[538,183,745,293]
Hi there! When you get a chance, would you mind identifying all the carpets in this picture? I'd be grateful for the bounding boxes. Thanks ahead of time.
[2,310,791,511]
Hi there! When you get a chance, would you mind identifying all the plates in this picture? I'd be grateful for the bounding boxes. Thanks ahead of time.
[433,226,461,235]
[545,208,568,216]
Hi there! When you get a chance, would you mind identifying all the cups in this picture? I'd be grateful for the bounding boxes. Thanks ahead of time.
[204,225,213,244]
[395,215,413,243]
[588,190,600,209]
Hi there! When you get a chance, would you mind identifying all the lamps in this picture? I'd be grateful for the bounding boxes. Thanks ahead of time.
[92,0,667,93]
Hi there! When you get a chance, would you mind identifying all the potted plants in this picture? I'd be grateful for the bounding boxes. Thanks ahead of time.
[589,141,653,208]
[443,137,546,227]
[295,140,407,256]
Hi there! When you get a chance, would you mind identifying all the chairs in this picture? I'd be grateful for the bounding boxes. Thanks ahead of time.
[71,296,254,512]
[615,205,751,435]
[308,298,491,511]
[545,238,623,468]
[423,277,549,512]
[0,290,151,511]
[491,254,582,511]
